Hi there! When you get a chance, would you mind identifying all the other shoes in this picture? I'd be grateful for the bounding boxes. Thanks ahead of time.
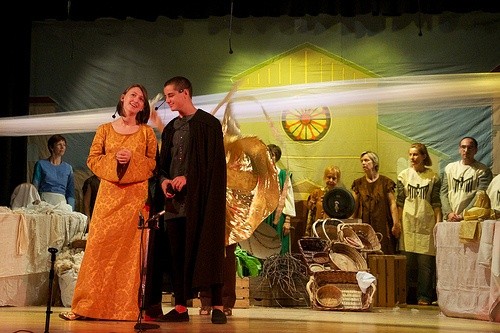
[418,298,428,305]
[200,304,211,315]
[223,308,232,316]
[58,311,88,320]
[432,300,439,305]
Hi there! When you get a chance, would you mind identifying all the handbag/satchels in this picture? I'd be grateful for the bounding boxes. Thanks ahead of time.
[234,245,262,279]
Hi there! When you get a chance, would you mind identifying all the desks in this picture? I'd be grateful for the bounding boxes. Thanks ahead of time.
[432,220,500,321]
[0,209,88,307]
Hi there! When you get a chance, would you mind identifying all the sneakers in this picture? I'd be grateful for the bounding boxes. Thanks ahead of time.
[156,308,189,322]
[212,308,227,323]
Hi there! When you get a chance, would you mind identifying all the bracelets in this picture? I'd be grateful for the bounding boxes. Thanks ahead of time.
[285,217,290,224]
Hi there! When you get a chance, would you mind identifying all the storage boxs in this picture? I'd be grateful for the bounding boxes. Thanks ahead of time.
[235,276,272,309]
[367,254,408,308]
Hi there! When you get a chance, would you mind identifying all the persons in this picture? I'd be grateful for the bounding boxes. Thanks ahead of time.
[262,144,297,255]
[59,84,157,320]
[306,166,341,235]
[144,76,238,324]
[439,137,493,222]
[33,133,75,210]
[350,143,442,305]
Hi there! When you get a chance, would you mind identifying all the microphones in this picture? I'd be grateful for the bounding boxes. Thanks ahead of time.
[155,100,165,110]
[112,111,117,118]
[138,212,143,227]
[147,210,165,222]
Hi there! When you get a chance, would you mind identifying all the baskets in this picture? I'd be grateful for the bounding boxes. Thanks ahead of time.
[298,237,337,274]
[313,218,383,254]
[306,271,376,312]
[316,286,343,308]
[329,240,368,270]
